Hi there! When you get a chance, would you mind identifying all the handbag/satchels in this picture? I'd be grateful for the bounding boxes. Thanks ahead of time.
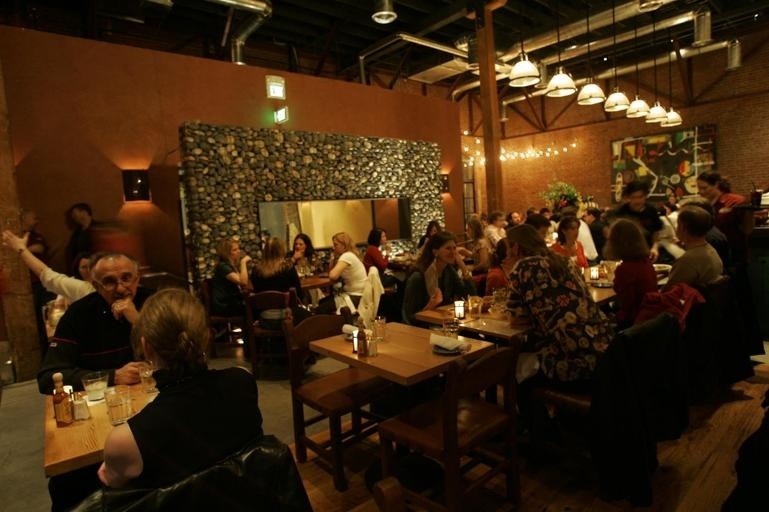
[293,255,297,261]
[290,257,295,265]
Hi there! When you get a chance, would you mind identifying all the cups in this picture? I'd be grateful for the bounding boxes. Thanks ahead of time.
[100,277,139,291]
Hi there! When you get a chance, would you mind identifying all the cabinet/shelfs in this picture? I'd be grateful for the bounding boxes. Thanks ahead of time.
[52,373,91,428]
[579,259,616,287]
[351,316,385,358]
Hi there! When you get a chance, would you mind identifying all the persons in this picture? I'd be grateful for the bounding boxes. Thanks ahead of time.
[457,213,493,274]
[504,223,610,446]
[675,197,730,271]
[483,208,556,248]
[697,175,750,250]
[362,227,399,292]
[582,207,605,262]
[547,216,589,268]
[318,232,368,316]
[70,285,264,486]
[2,199,188,397]
[485,238,518,295]
[416,221,443,246]
[662,205,723,290]
[606,180,665,263]
[249,236,315,366]
[606,218,659,331]
[403,231,477,327]
[285,233,317,273]
[551,194,598,265]
[209,238,253,360]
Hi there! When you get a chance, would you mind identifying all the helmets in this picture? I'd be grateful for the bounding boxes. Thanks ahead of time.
[431,346,460,354]
[653,264,671,272]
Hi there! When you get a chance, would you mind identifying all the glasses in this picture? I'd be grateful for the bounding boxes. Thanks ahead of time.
[505,1,684,129]
[121,167,151,202]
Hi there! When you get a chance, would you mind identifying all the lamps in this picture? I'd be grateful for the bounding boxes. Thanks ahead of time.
[505,1,684,129]
[121,167,151,202]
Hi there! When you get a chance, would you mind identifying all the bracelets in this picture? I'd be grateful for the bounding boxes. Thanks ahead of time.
[290,257,295,265]
[293,255,297,261]
[392,256,406,262]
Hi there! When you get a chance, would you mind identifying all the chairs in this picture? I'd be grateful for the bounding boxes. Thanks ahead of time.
[201,206,768,509]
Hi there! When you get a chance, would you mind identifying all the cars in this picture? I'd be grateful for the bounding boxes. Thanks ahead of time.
[140,360,159,394]
[81,370,109,401]
[42,298,66,329]
[104,385,133,426]
[442,319,459,339]
[294,264,311,282]
[750,190,762,206]
[465,289,507,321]
